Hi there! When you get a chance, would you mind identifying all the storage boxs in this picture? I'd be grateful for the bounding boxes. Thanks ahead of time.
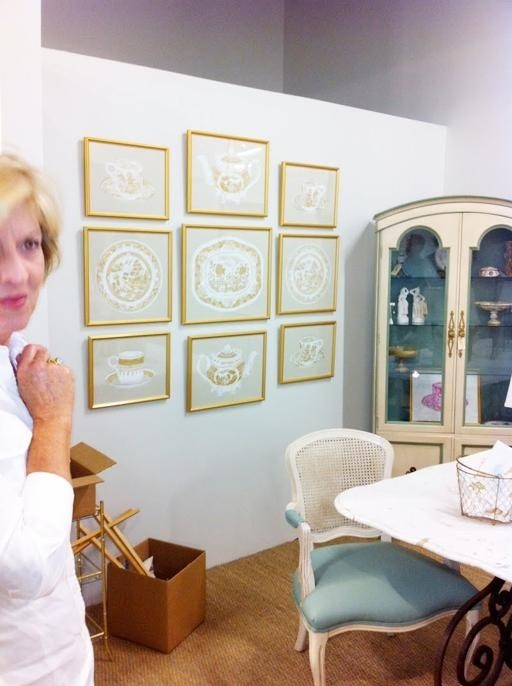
[106,537,207,654]
[70,441,117,520]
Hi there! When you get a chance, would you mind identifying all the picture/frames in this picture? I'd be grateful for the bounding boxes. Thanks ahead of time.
[83,225,173,325]
[83,135,169,221]
[277,233,340,316]
[186,330,268,413]
[279,159,340,229]
[409,369,482,425]
[279,320,337,384]
[181,222,273,326]
[186,128,270,217]
[87,330,171,411]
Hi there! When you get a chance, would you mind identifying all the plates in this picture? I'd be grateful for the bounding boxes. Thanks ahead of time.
[105,370,155,388]
[291,351,325,369]
[472,299,512,325]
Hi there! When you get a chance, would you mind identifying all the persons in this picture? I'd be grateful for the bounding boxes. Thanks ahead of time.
[0,149,96,686]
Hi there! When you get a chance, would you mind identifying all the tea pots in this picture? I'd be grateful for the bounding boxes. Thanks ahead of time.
[196,149,261,203]
[197,345,256,387]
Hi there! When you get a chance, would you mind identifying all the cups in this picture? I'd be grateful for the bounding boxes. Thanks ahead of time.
[109,351,143,384]
[300,336,322,364]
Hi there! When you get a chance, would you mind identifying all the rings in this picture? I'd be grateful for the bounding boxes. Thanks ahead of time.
[46,357,63,366]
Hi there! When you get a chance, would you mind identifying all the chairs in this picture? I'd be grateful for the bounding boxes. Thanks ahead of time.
[284,427,484,686]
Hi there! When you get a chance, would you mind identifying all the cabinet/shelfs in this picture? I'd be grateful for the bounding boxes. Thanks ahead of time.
[371,194,512,479]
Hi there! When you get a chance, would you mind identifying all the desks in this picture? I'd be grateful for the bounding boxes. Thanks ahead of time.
[333,460,512,686]
